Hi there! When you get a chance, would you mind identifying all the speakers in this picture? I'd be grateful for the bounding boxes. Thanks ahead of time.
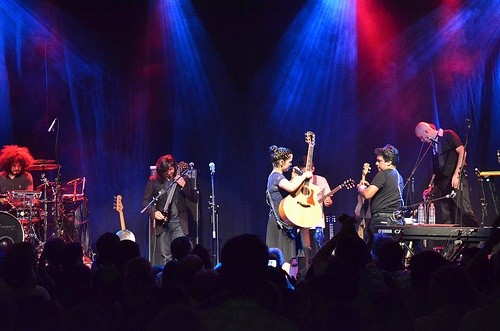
[149,176,198,266]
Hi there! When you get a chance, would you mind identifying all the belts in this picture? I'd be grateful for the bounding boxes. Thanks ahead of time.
[373,213,390,218]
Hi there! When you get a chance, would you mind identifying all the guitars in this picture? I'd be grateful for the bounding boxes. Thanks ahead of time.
[113,194,136,243]
[278,131,323,230]
[318,178,356,204]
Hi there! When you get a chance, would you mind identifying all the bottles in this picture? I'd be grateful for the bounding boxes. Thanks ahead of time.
[429,203,436,225]
[418,203,425,224]
[289,259,298,279]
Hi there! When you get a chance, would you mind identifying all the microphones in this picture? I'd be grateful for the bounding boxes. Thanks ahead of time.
[48,119,57,132]
[209,162,215,173]
[189,162,194,171]
[465,118,472,124]
[427,136,440,145]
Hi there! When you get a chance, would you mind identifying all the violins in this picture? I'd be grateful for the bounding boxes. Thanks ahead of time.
[354,163,371,219]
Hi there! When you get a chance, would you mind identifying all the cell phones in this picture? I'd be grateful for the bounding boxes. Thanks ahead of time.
[268,260,277,267]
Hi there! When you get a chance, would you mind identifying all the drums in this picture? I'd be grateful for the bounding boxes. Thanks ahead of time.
[16,208,43,224]
[0,211,25,247]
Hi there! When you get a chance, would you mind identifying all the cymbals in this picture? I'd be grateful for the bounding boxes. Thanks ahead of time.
[23,159,63,171]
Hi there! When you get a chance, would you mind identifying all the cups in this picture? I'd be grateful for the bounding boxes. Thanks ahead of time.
[404,218,416,225]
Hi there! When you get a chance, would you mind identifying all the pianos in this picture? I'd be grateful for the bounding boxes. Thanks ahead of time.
[372,223,500,243]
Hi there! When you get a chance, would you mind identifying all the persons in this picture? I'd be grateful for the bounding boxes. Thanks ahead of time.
[0,215,500,331]
[0,145,34,209]
[265,145,315,279]
[357,145,406,260]
[297,155,332,257]
[416,122,479,226]
[143,156,198,264]
